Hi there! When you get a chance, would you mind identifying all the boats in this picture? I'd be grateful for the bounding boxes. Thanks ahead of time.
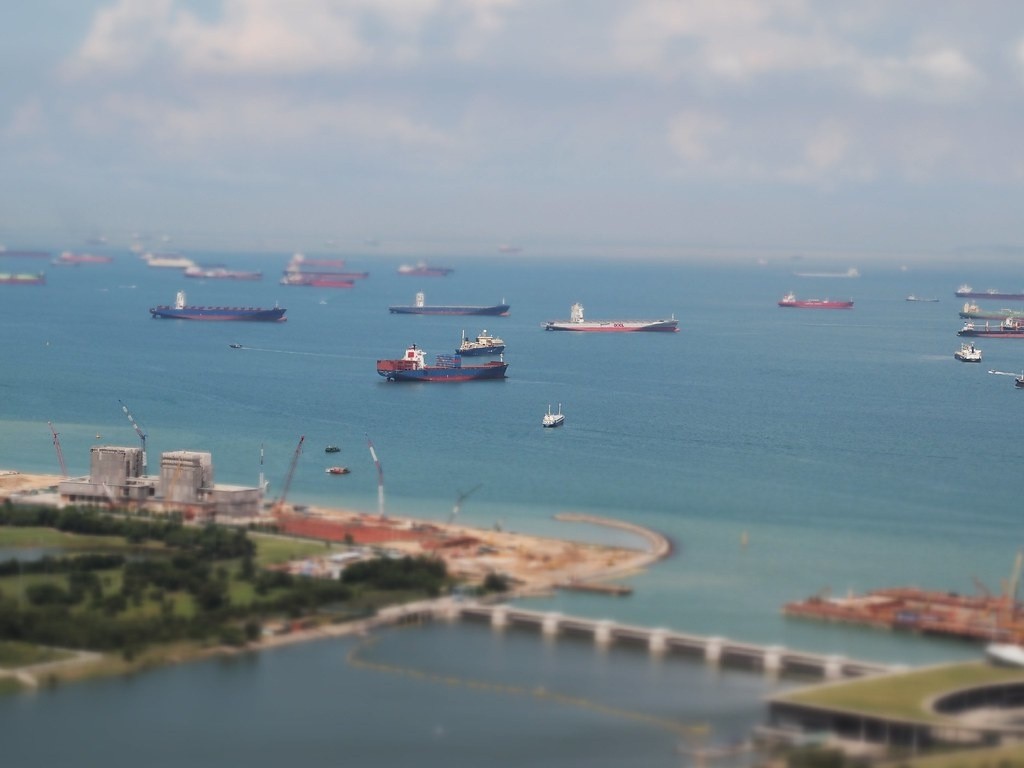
[229,344,241,349]
[185,267,262,279]
[989,369,1000,374]
[325,468,351,474]
[389,291,511,316]
[59,252,112,263]
[958,301,1024,319]
[150,289,288,320]
[0,271,48,285]
[958,315,1024,337]
[142,256,196,267]
[954,283,1024,300]
[1015,373,1024,383]
[797,267,860,277]
[778,293,855,308]
[294,255,345,268]
[954,341,981,362]
[905,294,939,302]
[0,248,52,258]
[455,327,506,356]
[376,342,509,380]
[326,446,341,452]
[540,302,680,332]
[281,268,369,287]
[542,403,566,427]
[399,259,454,276]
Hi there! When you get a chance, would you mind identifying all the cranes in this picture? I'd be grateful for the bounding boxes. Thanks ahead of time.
[258,442,271,508]
